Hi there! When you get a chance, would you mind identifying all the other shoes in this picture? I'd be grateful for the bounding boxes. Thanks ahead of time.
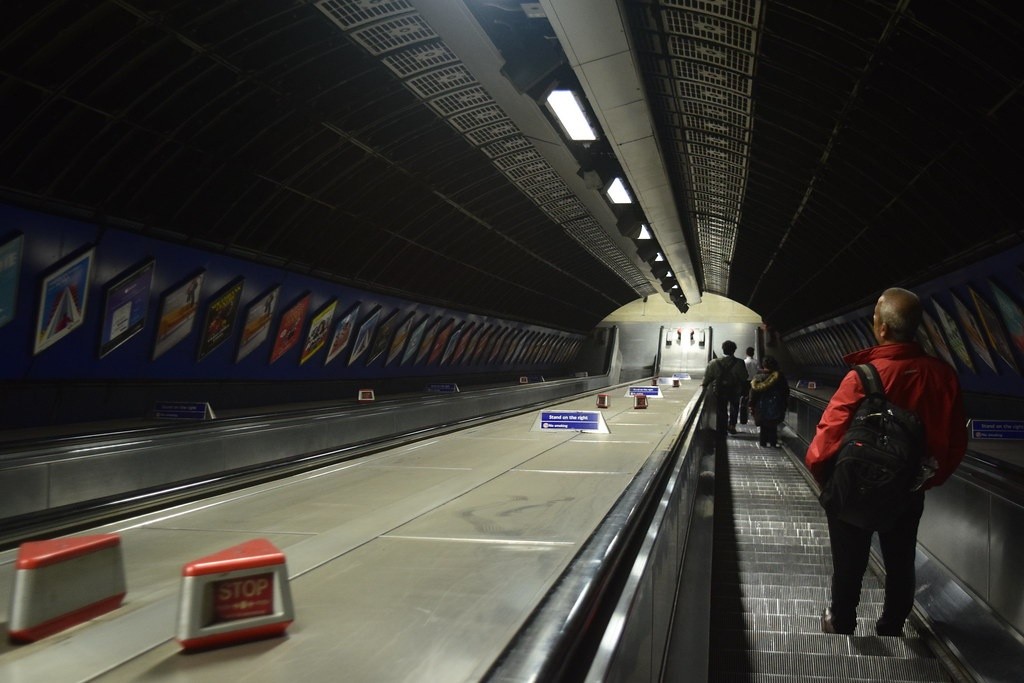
[822,607,836,633]
[728,424,737,433]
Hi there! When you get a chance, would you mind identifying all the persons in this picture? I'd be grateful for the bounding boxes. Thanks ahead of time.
[805,288,966,637]
[699,340,787,448]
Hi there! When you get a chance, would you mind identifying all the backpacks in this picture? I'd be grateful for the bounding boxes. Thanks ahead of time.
[716,357,740,399]
[819,362,926,537]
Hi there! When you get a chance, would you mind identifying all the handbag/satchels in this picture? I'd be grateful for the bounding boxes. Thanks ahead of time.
[752,398,783,426]
